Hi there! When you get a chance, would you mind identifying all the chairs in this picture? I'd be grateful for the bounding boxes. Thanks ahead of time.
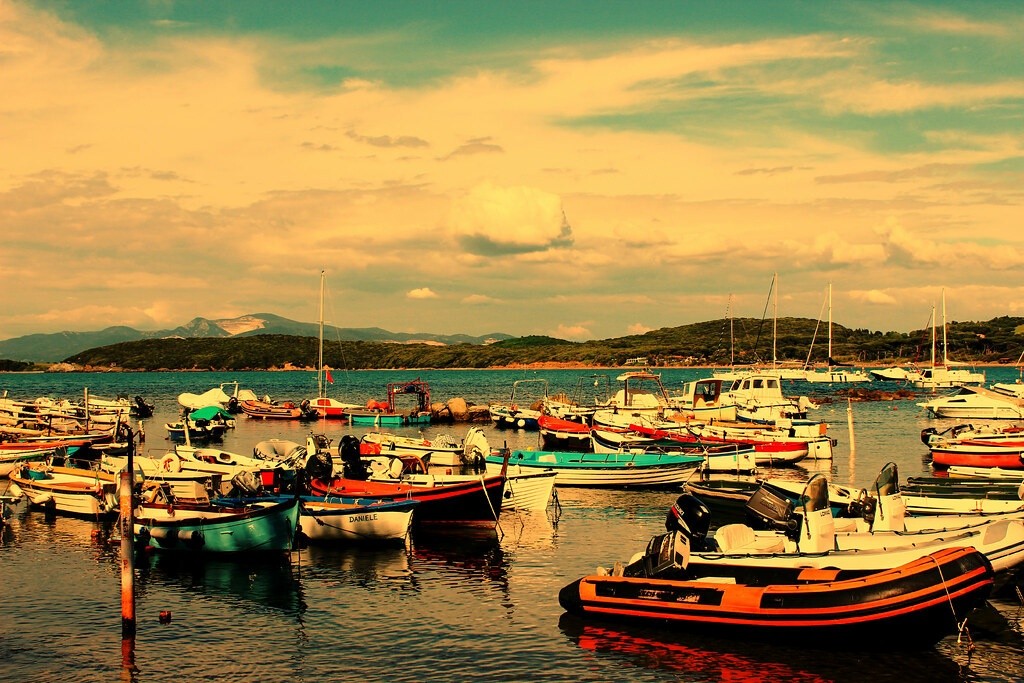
[714,524,785,555]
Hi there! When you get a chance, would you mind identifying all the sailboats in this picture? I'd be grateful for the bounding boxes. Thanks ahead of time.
[801,284,871,383]
[905,287,984,388]
[306,270,364,415]
[711,273,815,378]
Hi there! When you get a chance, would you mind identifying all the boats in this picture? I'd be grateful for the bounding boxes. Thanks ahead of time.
[490,370,833,473]
[0,386,155,461]
[556,533,995,651]
[483,442,700,486]
[915,394,1024,420]
[102,438,309,504]
[133,496,299,565]
[870,363,920,381]
[164,382,317,441]
[900,424,1024,500]
[628,463,1024,578]
[993,382,1024,398]
[342,408,430,427]
[228,473,420,550]
[8,453,119,516]
[303,426,557,533]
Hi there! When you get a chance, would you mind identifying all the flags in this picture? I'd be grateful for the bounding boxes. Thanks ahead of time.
[325,369,334,385]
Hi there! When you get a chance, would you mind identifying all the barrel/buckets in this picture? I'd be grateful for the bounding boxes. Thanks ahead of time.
[21,468,44,480]
